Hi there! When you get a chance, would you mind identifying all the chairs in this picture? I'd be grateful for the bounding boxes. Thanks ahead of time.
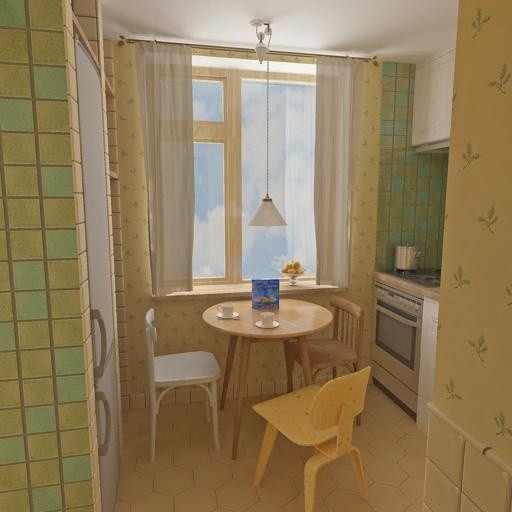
[250,364,377,512]
[300,295,366,427]
[141,308,225,464]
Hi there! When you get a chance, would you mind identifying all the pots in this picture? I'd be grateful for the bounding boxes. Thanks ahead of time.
[393,240,422,272]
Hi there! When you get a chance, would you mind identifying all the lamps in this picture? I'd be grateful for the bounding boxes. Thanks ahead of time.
[247,18,289,229]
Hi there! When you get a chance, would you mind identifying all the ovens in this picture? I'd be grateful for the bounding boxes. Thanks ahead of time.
[372,281,423,421]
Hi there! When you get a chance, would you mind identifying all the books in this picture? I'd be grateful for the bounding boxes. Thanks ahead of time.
[250,278,281,309]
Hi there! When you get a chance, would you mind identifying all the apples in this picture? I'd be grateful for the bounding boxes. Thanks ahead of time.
[282,261,304,273]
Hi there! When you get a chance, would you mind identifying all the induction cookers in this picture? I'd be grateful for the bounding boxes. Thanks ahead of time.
[376,267,441,294]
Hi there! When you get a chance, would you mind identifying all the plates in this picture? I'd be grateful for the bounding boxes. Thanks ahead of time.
[216,312,240,319]
[254,321,280,329]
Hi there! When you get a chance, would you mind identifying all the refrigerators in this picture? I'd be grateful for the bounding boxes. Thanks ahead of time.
[71,33,123,511]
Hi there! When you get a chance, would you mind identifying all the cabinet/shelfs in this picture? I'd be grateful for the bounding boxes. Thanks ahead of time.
[416,296,439,439]
[408,48,456,148]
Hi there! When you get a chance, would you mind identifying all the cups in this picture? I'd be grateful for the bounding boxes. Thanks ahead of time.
[216,302,233,316]
[259,311,274,326]
